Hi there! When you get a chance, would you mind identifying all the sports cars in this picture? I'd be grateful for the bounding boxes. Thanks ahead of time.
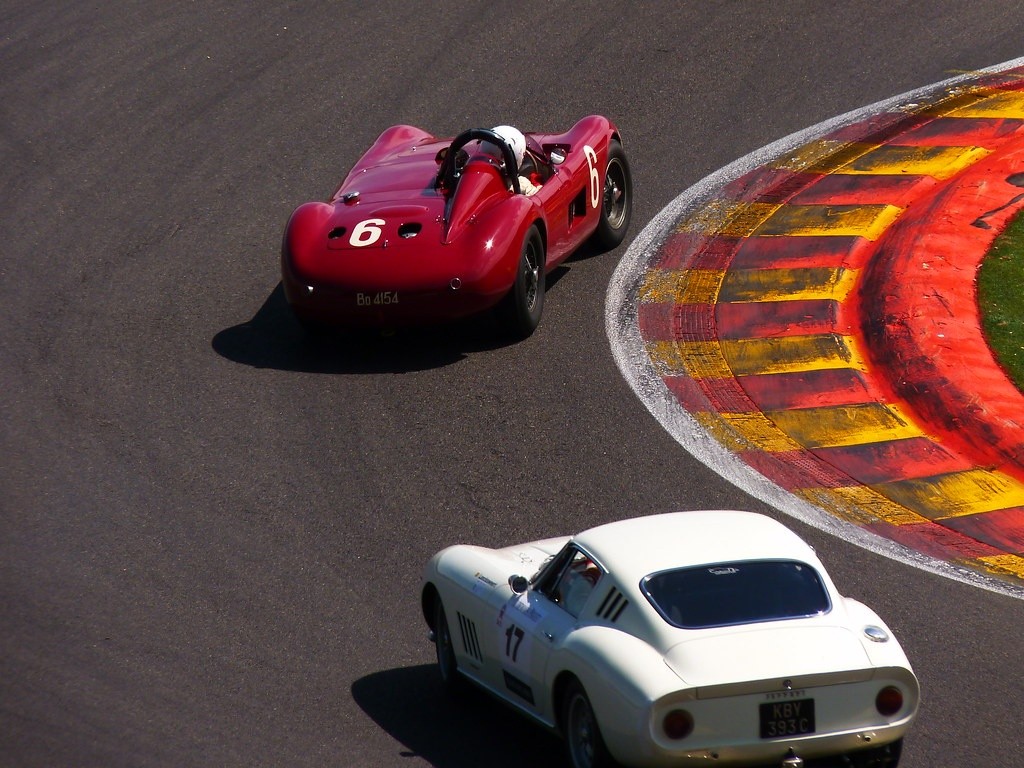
[424,508,920,768]
[283,115,632,336]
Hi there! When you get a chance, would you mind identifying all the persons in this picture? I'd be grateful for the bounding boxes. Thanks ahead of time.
[478,125,543,197]
[558,556,603,609]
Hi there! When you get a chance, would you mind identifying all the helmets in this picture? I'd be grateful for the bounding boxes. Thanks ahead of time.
[480,124,526,182]
